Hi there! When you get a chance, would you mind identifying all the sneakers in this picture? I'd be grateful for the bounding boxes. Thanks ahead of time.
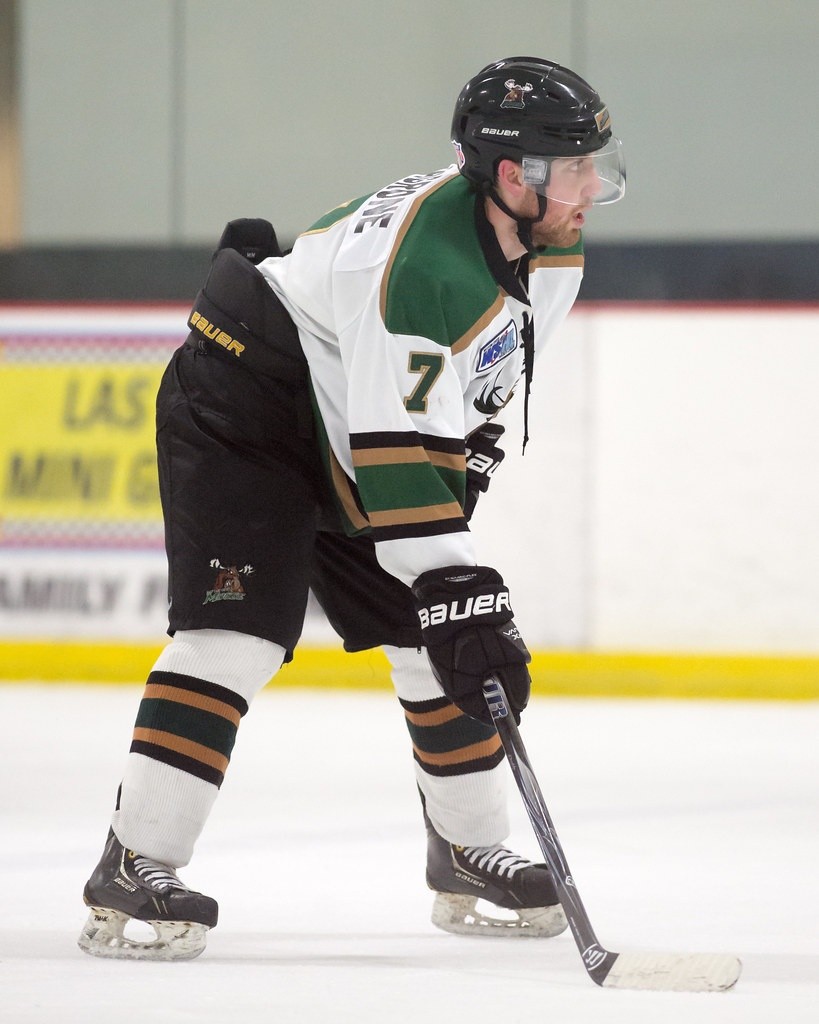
[422,822,568,938]
[74,824,219,960]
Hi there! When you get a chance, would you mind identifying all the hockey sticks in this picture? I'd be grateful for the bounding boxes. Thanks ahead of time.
[465,678,747,996]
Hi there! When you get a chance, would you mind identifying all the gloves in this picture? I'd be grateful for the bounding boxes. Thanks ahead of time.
[456,434,504,522]
[409,562,533,728]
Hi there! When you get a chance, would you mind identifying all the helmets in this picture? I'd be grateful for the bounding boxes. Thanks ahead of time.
[449,56,613,189]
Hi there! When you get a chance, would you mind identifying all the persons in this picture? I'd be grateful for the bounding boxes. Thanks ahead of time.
[76,57,628,958]
